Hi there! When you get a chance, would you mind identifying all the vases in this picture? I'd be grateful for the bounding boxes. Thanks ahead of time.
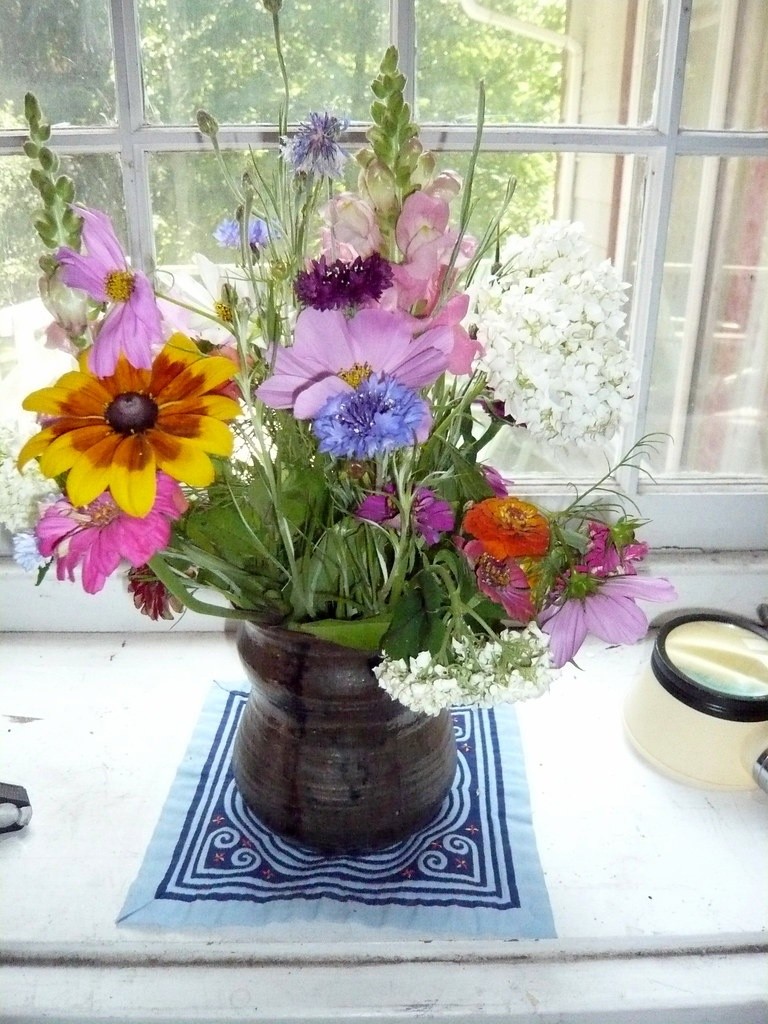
[233,611,458,853]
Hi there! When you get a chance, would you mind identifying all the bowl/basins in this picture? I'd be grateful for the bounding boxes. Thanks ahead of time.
[626,613,768,789]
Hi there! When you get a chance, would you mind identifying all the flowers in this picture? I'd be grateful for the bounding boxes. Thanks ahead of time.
[0,0,675,716]
